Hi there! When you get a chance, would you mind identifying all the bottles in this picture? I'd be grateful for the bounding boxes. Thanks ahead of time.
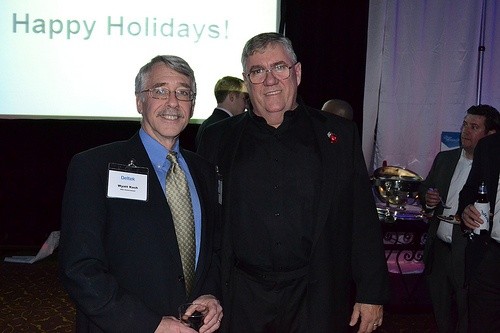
[472,177,490,237]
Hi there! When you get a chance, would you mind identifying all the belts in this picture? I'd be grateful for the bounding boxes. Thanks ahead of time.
[490,238,500,247]
[434,237,451,247]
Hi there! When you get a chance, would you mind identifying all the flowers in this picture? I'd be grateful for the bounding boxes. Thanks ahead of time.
[327,131,337,142]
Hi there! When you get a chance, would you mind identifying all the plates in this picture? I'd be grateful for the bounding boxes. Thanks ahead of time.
[437,215,461,225]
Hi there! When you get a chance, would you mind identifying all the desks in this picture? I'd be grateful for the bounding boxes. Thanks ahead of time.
[377,212,430,299]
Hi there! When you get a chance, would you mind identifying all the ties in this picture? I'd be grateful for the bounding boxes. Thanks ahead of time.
[166,151,196,299]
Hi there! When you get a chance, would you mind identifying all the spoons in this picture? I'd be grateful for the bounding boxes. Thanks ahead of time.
[430,187,451,210]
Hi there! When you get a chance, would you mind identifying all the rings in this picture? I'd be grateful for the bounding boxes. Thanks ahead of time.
[374,324,378,326]
[218,320,220,322]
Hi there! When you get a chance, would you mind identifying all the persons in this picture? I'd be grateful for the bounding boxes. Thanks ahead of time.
[62,56,228,333]
[416,104,500,333]
[212,33,386,333]
[196,77,248,169]
[321,99,353,121]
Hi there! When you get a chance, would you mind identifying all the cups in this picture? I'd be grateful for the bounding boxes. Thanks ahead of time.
[178,303,209,332]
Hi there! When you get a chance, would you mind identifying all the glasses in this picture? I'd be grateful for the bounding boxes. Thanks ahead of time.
[137,86,195,101]
[245,62,296,84]
